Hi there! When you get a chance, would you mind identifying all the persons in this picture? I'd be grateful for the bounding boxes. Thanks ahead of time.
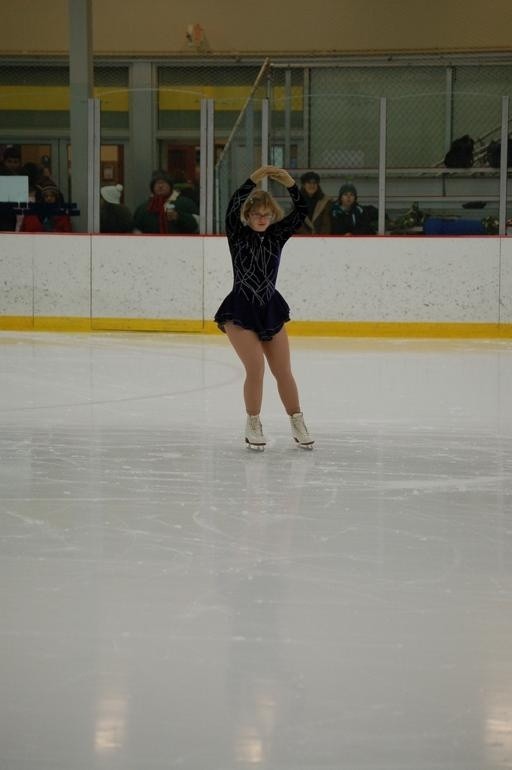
[291,172,365,235]
[0,148,71,232]
[133,169,199,234]
[101,184,132,233]
[215,166,315,446]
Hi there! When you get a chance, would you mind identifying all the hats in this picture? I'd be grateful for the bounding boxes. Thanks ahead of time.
[100,184,123,205]
[339,184,358,201]
[36,175,59,203]
[150,172,173,193]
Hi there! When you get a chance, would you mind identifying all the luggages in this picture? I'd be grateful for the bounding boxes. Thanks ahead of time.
[444,135,474,168]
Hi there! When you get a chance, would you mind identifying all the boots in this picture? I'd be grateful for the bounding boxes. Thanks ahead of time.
[290,413,314,447]
[245,414,267,446]
